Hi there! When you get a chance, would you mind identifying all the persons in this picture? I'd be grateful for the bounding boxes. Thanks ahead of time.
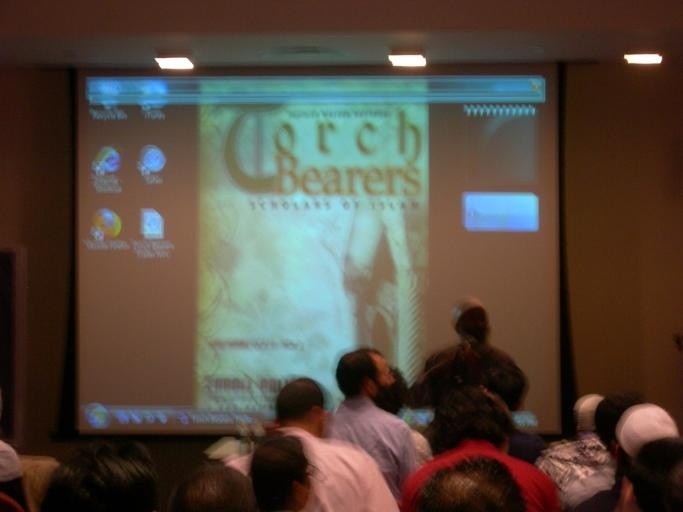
[174,351,683,512]
[0,440,163,512]
[402,298,530,419]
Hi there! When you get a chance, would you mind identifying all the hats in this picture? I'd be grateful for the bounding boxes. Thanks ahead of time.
[614,402,680,459]
[0,439,25,482]
